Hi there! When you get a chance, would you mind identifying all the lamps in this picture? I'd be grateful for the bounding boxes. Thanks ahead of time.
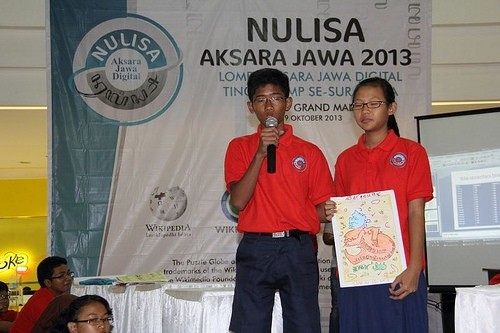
[16,266,28,305]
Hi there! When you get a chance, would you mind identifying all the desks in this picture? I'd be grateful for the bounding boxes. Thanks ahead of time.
[71,280,283,333]
[454,283,500,333]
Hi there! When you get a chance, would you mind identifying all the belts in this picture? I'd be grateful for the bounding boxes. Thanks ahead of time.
[245,231,309,238]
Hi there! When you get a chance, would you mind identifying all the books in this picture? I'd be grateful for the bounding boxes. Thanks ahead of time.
[330,189,409,289]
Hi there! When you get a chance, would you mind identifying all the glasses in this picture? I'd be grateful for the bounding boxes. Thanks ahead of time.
[0,295,10,300]
[350,101,389,110]
[76,316,113,325]
[253,97,286,104]
[51,271,74,280]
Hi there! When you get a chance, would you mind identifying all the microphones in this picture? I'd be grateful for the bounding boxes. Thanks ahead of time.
[266,115,279,173]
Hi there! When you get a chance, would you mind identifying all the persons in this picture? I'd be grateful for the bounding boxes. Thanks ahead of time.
[0,281,20,333]
[49,295,114,333]
[318,77,434,333]
[224,67,336,333]
[8,256,74,333]
